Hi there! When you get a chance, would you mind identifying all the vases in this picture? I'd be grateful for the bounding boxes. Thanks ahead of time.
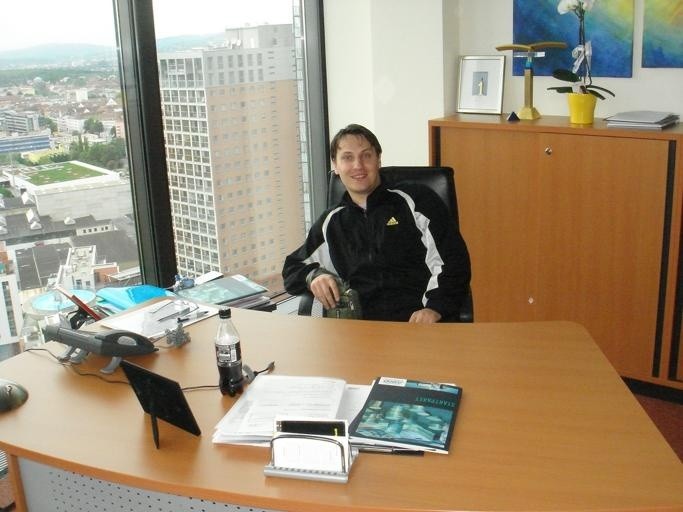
[564,93,597,125]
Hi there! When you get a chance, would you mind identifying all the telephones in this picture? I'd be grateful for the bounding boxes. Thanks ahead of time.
[42,325,159,373]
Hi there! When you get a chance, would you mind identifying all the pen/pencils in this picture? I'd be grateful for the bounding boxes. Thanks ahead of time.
[357,446,424,455]
[157,307,208,323]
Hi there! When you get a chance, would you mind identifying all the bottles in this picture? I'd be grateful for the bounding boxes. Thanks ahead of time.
[212,308,244,398]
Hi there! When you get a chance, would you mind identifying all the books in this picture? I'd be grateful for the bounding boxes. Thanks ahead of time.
[352,377,464,455]
[172,273,272,307]
[602,110,681,130]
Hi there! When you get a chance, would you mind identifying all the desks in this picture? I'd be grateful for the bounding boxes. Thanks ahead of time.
[1,298,683,511]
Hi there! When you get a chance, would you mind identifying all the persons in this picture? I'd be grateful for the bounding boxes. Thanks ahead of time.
[281,124,472,325]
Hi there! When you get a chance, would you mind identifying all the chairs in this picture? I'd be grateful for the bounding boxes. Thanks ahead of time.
[297,165,474,322]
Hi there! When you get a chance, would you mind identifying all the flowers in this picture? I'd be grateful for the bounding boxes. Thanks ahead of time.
[546,0,616,100]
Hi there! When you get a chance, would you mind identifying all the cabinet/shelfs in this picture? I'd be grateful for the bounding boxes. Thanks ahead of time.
[428,112,683,392]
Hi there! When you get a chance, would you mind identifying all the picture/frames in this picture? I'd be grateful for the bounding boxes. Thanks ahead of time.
[119,359,201,448]
[456,55,506,115]
